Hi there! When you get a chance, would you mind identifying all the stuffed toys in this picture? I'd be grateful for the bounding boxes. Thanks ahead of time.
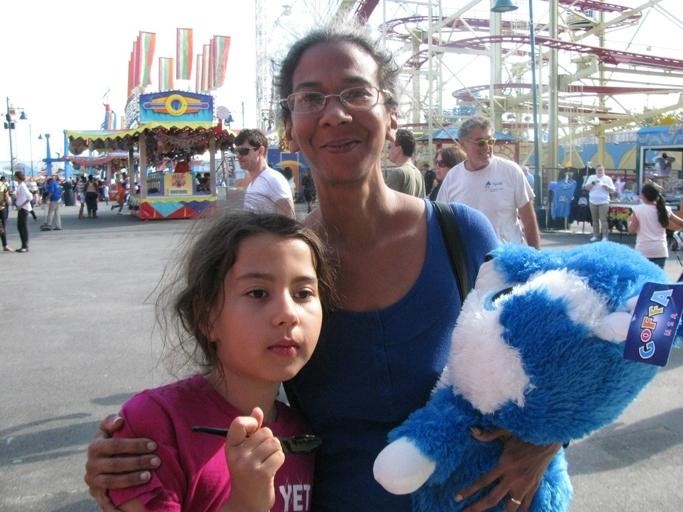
[372,240,683,512]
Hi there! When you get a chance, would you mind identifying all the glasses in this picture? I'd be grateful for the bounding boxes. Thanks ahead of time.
[459,135,495,145]
[434,159,447,168]
[234,148,258,155]
[280,86,396,111]
[388,143,402,149]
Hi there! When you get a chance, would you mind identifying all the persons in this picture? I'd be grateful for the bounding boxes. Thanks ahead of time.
[383,128,426,200]
[103,185,110,207]
[1,179,12,252]
[428,146,468,198]
[121,171,129,187]
[62,178,73,206]
[40,175,62,231]
[134,181,141,196]
[84,175,98,219]
[12,171,33,252]
[102,177,109,187]
[110,182,127,213]
[27,178,38,208]
[235,128,295,220]
[435,116,542,251]
[627,182,683,270]
[85,28,563,512]
[0,175,10,191]
[107,197,343,512]
[583,163,616,243]
[73,176,88,220]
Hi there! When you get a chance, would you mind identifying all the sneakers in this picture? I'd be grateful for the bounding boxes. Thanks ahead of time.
[601,237,609,242]
[3,245,12,251]
[589,235,600,242]
[16,247,28,252]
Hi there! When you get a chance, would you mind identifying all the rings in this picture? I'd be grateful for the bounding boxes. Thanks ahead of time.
[510,496,522,506]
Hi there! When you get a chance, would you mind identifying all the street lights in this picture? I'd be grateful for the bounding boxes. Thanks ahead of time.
[491,0,548,228]
[6,96,28,192]
[8,97,29,194]
